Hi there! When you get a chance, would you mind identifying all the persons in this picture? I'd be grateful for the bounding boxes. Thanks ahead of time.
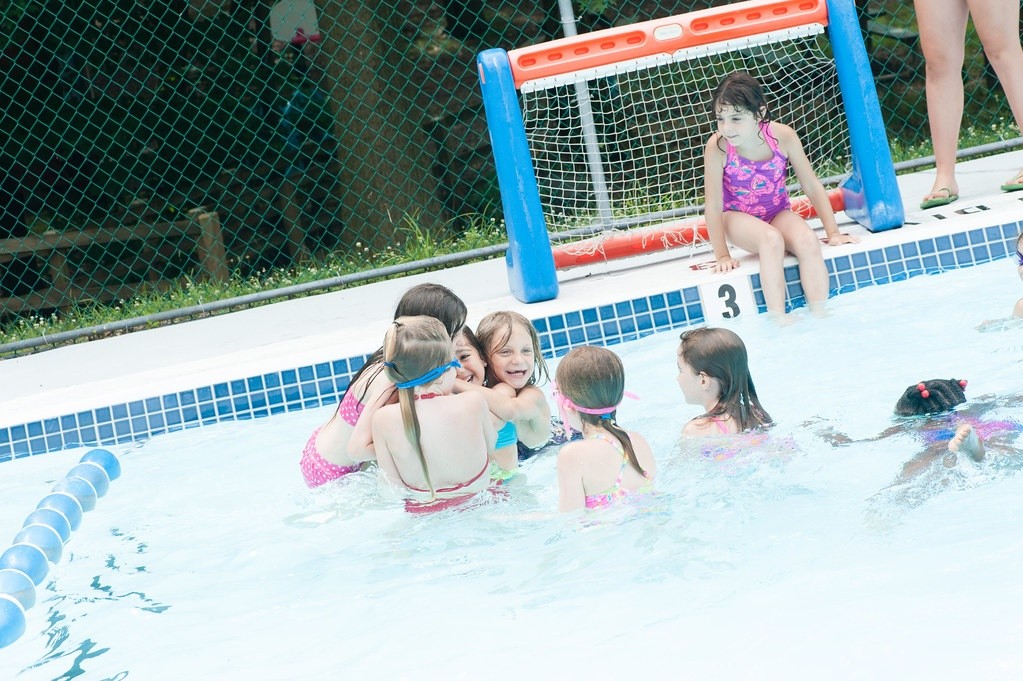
[822,373,1022,486]
[676,327,776,438]
[914,0,1022,209]
[1010,231,1023,322]
[703,72,860,320]
[552,346,661,513]
[300,281,581,515]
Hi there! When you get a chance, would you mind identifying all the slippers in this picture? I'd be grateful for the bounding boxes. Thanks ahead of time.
[920,187,959,209]
[1001,175,1023,190]
